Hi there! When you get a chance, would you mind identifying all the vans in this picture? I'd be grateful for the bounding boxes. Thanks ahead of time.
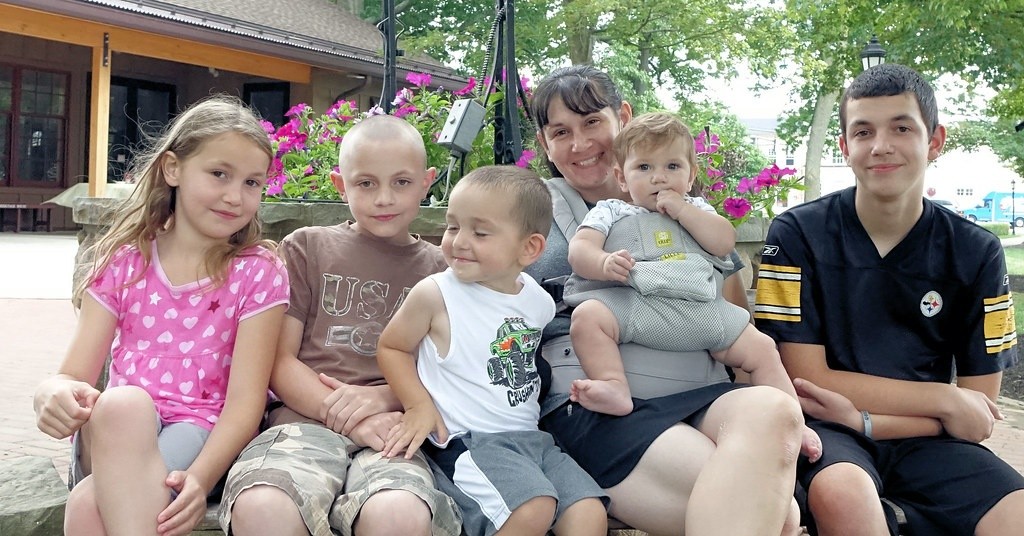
[962,189,1024,229]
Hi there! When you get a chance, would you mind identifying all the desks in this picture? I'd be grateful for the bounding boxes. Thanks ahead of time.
[0,201,56,234]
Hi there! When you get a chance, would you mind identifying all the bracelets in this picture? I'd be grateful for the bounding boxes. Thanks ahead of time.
[861,410,873,439]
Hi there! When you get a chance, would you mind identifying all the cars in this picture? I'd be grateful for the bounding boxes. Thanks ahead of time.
[929,198,962,215]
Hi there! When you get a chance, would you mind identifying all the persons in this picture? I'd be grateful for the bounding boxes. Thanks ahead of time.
[377,164,609,536]
[756,63,1024,536]
[520,66,822,536]
[219,115,449,536]
[34,96,290,536]
